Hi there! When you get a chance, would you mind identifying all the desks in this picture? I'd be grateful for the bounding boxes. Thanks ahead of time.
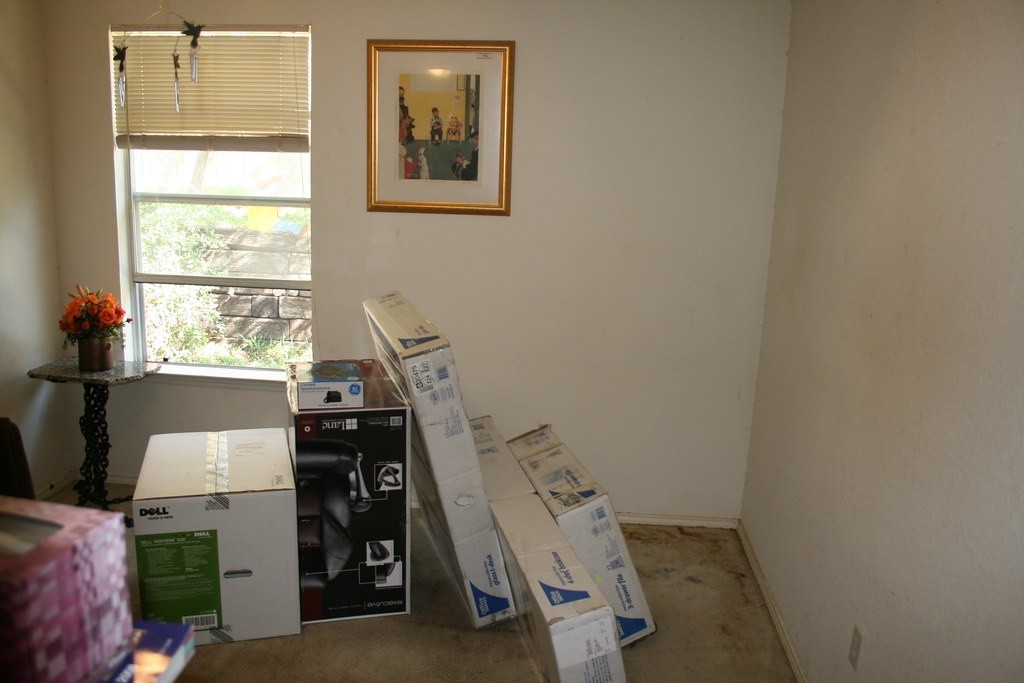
[27,356,163,525]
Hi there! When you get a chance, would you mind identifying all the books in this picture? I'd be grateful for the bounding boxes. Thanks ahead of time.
[100,620,197,683]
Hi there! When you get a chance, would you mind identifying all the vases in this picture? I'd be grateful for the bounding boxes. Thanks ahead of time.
[77,337,113,371]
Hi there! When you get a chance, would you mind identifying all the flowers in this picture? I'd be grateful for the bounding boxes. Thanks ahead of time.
[57,282,132,353]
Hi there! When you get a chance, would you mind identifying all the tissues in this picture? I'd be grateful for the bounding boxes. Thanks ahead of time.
[0,492,135,683]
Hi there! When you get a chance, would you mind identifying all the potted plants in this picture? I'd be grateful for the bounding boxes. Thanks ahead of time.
[114,18,206,115]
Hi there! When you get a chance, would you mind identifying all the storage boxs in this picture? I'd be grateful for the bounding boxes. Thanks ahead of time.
[363,291,517,628]
[0,497,136,683]
[507,422,656,648]
[470,414,627,683]
[286,357,410,625]
[133,427,301,645]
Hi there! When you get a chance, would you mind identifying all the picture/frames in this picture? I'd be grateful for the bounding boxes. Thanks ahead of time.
[364,38,514,216]
